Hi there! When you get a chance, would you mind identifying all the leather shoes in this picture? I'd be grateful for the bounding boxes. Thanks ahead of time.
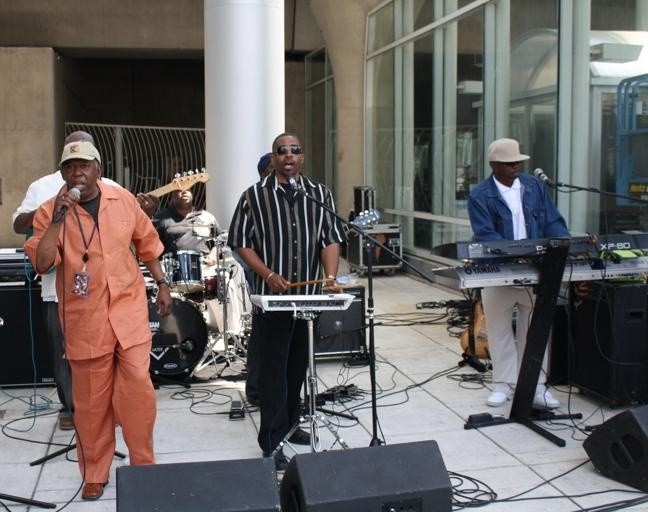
[82,477,109,500]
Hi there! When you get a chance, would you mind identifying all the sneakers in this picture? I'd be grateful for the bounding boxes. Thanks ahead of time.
[59,416,76,431]
[486,386,513,407]
[532,388,559,409]
[284,425,319,445]
[262,446,289,475]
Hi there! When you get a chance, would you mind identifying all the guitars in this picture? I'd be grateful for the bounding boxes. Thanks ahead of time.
[459,298,491,358]
[26,167,209,274]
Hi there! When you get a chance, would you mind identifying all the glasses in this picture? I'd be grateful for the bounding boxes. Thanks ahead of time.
[273,146,303,156]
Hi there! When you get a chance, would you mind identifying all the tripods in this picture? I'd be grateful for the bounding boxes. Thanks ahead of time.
[30,439,125,468]
[268,315,351,458]
[188,224,247,380]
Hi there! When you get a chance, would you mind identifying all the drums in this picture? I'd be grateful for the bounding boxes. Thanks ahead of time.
[147,294,208,377]
[203,276,218,301]
[163,251,203,294]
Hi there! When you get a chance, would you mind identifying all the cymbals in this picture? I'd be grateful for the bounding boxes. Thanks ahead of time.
[171,221,212,228]
[193,234,227,242]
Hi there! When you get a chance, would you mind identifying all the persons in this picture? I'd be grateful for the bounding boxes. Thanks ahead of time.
[12,132,159,431]
[469,138,573,408]
[232,153,277,405]
[25,140,174,501]
[157,188,245,351]
[227,132,343,469]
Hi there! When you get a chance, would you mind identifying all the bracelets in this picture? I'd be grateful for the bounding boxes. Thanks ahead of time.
[264,270,276,283]
[326,274,339,280]
[157,278,167,287]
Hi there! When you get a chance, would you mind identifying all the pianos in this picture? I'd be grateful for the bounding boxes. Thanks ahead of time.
[431,230,646,289]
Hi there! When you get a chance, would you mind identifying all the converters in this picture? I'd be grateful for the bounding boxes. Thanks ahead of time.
[229,400,244,419]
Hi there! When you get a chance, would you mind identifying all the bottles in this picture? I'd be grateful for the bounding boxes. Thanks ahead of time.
[357,206,380,230]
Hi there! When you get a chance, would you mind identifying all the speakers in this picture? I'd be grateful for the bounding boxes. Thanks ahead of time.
[116,457,281,511]
[280,439,454,512]
[583,405,648,493]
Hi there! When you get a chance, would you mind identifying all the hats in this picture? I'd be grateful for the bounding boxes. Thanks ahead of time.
[489,137,530,164]
[57,141,102,170]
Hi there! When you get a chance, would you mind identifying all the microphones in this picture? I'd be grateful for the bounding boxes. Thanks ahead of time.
[53,187,81,224]
[533,168,556,189]
[286,172,299,192]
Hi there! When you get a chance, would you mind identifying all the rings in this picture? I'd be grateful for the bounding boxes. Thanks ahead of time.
[145,201,149,205]
